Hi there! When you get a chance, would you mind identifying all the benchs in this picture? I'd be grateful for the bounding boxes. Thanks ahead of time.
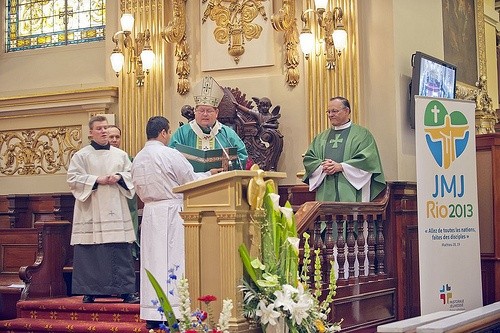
[0,221,72,320]
[0,193,74,271]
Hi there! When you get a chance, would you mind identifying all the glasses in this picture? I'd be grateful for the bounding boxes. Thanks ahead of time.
[326,109,345,115]
[197,109,215,115]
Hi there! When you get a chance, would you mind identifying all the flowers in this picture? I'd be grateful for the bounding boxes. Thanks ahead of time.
[238,182,345,333]
[145,264,233,333]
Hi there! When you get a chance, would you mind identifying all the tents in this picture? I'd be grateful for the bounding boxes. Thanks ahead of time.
[176,142,238,172]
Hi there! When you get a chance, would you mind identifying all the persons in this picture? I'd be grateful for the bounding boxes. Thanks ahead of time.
[303,95,387,245]
[67,116,140,304]
[168,76,248,172]
[130,116,224,329]
[18,130,41,174]
[108,124,138,259]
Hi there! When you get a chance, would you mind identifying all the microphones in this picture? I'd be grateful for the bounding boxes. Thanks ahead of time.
[218,129,246,174]
[207,124,234,172]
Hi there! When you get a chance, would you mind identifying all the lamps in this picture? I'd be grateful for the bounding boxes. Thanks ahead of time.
[300,0,347,70]
[110,14,156,86]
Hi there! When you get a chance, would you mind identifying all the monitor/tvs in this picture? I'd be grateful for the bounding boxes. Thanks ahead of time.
[410,51,459,133]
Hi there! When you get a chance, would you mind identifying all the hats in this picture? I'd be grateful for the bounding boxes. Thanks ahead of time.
[190,76,224,108]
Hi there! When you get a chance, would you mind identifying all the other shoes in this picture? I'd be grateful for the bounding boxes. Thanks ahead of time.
[123,294,140,304]
[145,319,168,329]
[83,295,94,303]
